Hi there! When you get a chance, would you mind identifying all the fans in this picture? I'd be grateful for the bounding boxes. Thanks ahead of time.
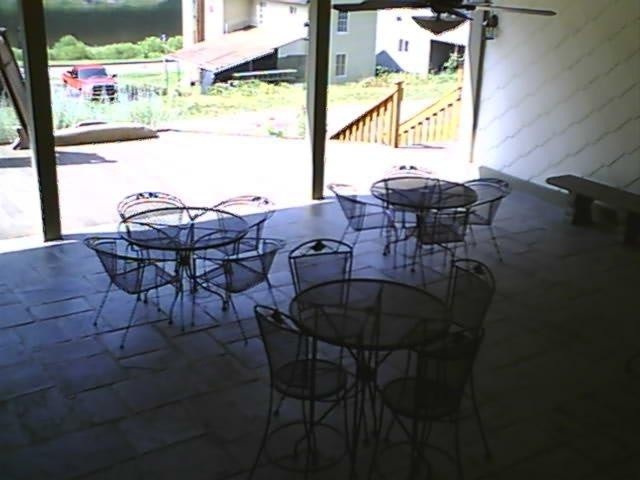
[329,0,557,34]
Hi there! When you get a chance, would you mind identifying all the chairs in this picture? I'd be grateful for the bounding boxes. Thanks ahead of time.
[83,188,287,350]
[326,165,511,287]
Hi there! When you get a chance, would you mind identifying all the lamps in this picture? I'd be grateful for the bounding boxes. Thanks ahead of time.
[482,10,500,38]
[410,12,467,36]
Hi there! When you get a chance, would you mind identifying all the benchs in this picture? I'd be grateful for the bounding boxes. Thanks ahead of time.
[546,174,639,243]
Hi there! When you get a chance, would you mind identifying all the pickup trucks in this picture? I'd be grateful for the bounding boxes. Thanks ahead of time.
[62,64,118,102]
